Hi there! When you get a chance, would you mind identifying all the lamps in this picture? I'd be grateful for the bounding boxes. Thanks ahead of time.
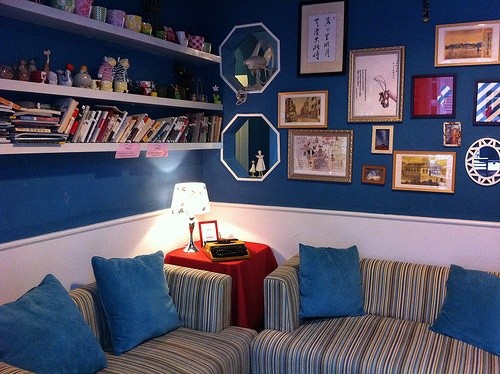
[170,180,213,255]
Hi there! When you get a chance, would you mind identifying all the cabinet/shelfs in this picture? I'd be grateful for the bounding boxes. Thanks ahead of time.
[0,0,225,155]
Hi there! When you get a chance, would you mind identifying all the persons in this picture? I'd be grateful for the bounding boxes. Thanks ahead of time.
[249,160,255,176]
[255,151,266,176]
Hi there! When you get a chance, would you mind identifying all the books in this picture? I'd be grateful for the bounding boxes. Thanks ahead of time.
[0,96,69,143]
[58,97,138,142]
[128,112,222,142]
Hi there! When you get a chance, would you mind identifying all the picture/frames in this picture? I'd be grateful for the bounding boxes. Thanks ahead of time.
[441,121,462,148]
[410,72,457,119]
[287,128,355,184]
[295,0,349,80]
[433,20,500,68]
[371,124,395,154]
[361,165,386,186]
[471,79,500,127]
[197,219,220,249]
[391,150,458,194]
[277,89,329,128]
[346,44,406,124]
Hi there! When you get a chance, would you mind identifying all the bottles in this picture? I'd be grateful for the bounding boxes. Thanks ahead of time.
[175,85,182,100]
[179,72,186,99]
[150,82,157,96]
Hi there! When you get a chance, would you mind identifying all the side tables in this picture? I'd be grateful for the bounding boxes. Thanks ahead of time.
[166,242,278,331]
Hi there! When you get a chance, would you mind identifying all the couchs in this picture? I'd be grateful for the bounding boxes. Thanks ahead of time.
[247,249,500,374]
[0,262,257,374]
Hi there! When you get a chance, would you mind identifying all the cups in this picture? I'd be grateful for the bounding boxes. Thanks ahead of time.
[188,36,204,51]
[202,43,212,54]
[107,10,126,28]
[142,23,152,35]
[50,0,94,18]
[156,31,178,44]
[126,15,142,33]
[176,32,190,47]
[91,6,107,23]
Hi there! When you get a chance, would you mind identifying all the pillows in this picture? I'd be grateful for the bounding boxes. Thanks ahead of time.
[0,273,108,374]
[430,263,500,358]
[295,242,364,320]
[89,252,182,355]
